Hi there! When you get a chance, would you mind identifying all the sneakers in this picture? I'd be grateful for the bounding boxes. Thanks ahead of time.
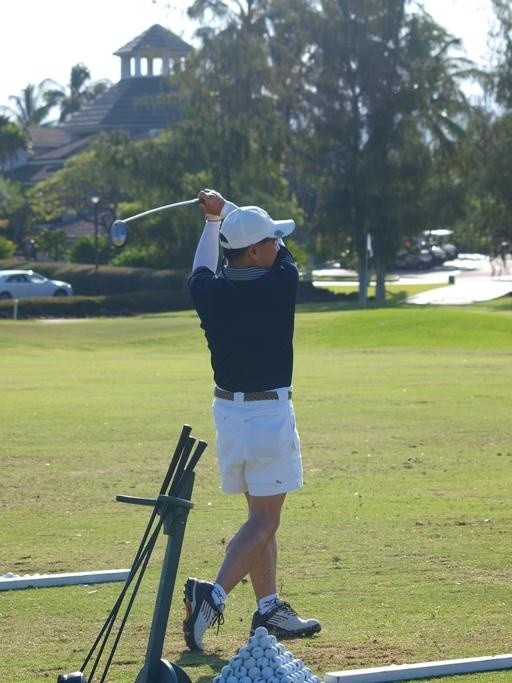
[249,600,323,644]
[180,578,226,653]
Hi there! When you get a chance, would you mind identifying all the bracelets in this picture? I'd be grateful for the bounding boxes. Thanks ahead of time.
[204,213,223,220]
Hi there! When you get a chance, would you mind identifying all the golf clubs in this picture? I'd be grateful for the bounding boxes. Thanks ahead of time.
[110,198,203,247]
[58,441,208,683]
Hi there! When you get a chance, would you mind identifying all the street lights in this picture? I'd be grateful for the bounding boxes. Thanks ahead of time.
[91,192,99,264]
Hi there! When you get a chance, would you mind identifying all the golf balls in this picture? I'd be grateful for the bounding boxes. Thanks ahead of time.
[213,627,320,683]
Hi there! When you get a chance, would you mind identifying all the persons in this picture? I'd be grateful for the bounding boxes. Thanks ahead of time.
[179,186,323,652]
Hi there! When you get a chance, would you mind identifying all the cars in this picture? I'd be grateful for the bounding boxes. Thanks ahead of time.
[0,269,74,300]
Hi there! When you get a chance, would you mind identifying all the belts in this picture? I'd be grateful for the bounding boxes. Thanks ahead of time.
[214,387,294,406]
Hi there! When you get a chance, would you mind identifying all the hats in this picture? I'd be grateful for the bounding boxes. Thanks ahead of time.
[218,204,297,253]
